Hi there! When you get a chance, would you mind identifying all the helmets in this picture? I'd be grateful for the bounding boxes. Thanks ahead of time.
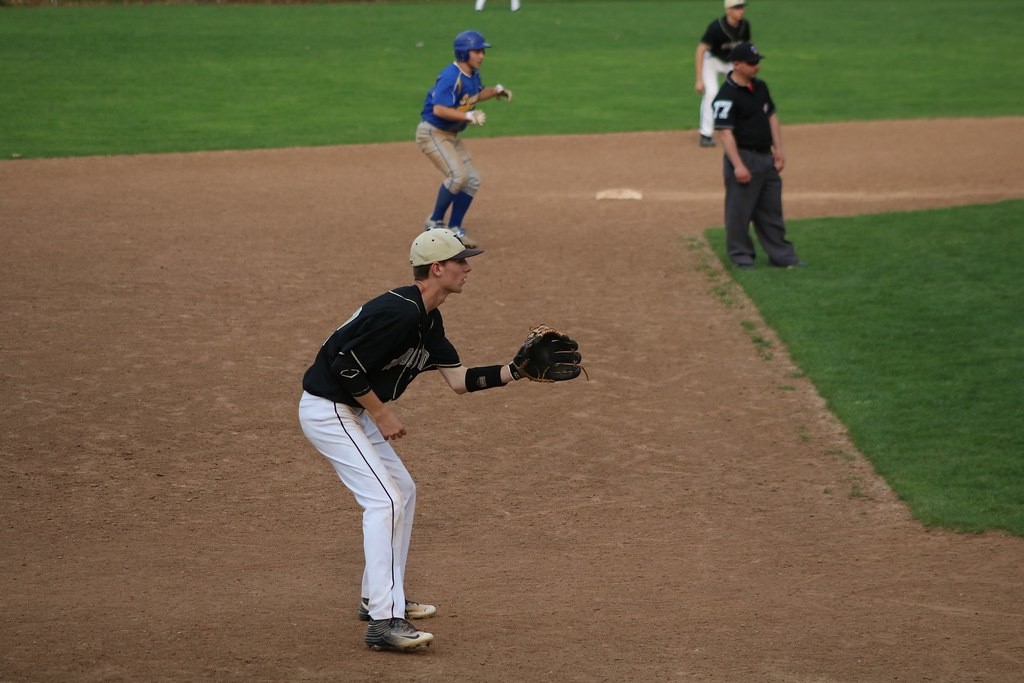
[454,30,491,61]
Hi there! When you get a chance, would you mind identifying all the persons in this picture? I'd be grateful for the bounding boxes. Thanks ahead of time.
[694,0,752,147]
[474,0,522,12]
[294,226,583,653]
[414,29,514,250]
[708,42,805,273]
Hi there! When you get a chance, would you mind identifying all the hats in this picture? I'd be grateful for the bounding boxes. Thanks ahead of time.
[731,43,765,64]
[410,229,485,266]
[724,0,746,7]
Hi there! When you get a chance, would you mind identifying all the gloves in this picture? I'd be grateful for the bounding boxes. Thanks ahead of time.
[495,84,512,102]
[467,110,486,126]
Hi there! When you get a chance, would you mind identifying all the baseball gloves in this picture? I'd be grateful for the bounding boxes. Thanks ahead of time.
[511,325,582,384]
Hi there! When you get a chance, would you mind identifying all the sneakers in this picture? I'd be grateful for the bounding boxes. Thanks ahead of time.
[359,596,436,620]
[365,617,434,649]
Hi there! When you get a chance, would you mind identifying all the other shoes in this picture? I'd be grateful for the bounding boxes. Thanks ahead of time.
[701,136,716,147]
[792,260,808,268]
[451,227,479,247]
[424,214,446,231]
